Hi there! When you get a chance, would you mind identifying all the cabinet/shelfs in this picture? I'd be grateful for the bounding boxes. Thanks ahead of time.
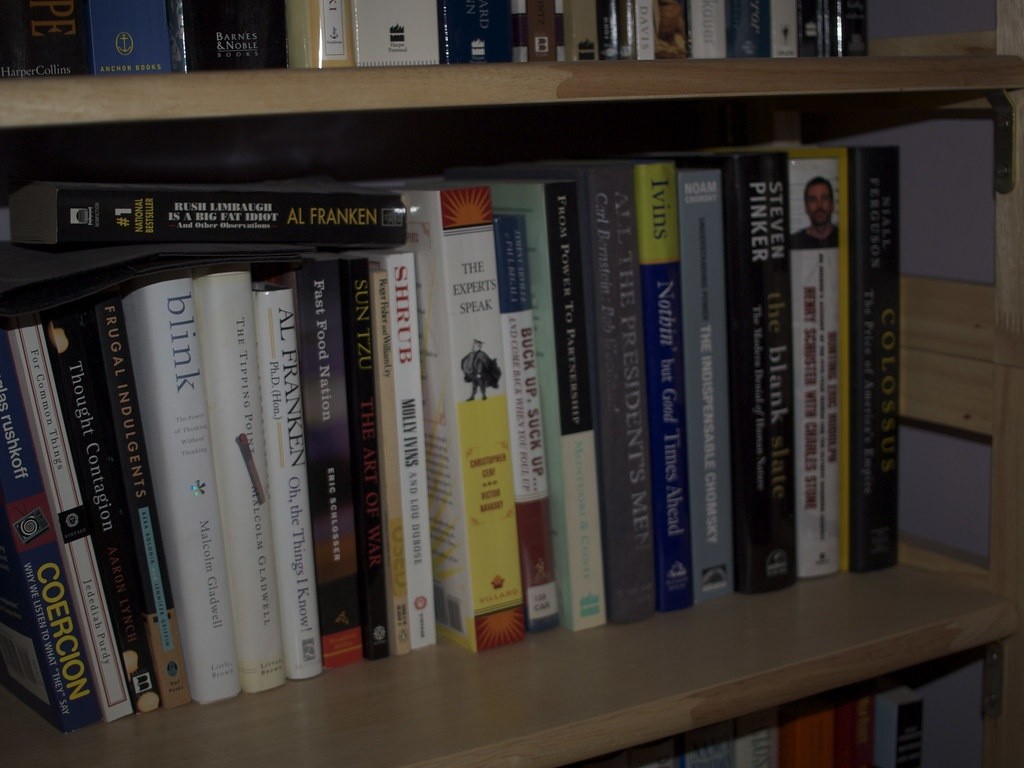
[0,0,1024,768]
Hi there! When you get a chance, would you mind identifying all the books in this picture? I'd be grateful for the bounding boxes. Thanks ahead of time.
[0,146,902,731]
[577,678,924,768]
[0,0,871,80]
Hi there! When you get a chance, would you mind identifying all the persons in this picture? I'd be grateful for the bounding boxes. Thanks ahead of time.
[792,176,838,249]
